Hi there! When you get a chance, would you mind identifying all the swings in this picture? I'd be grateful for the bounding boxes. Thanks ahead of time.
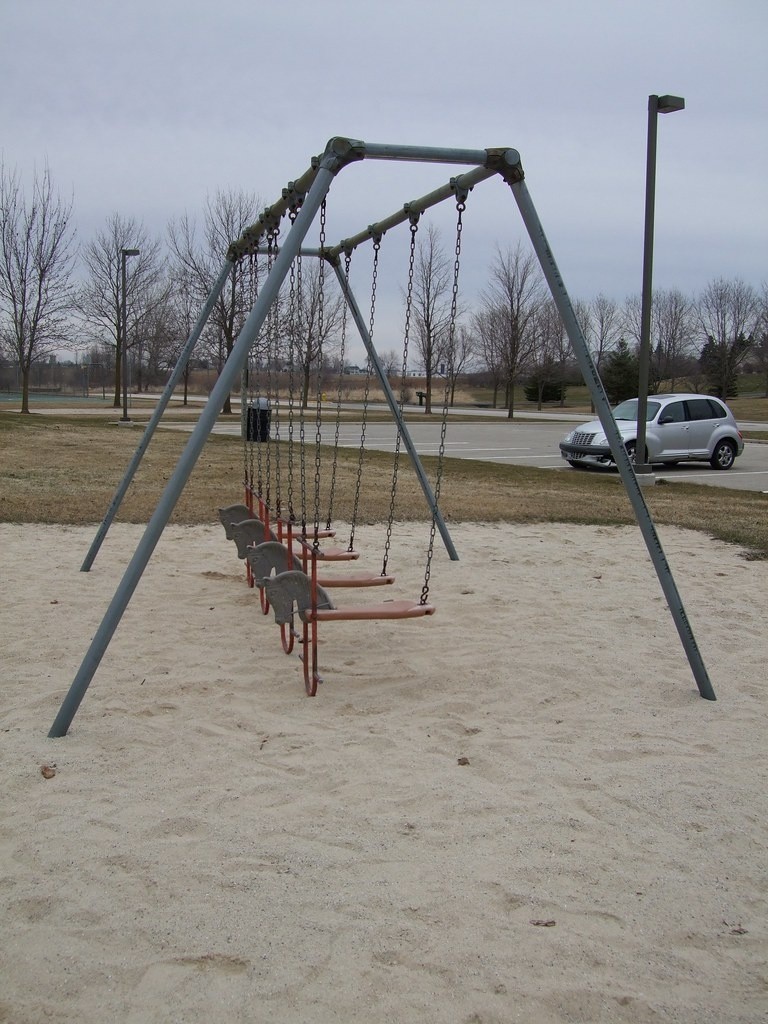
[217,204,467,697]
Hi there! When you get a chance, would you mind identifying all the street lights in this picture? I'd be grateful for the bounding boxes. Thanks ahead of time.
[635,94,686,473]
[122,248,139,422]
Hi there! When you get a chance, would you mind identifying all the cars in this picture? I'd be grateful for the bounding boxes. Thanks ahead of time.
[556,393,743,471]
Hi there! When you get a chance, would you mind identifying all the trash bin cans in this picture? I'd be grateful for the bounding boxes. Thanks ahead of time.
[247,396,272,442]
